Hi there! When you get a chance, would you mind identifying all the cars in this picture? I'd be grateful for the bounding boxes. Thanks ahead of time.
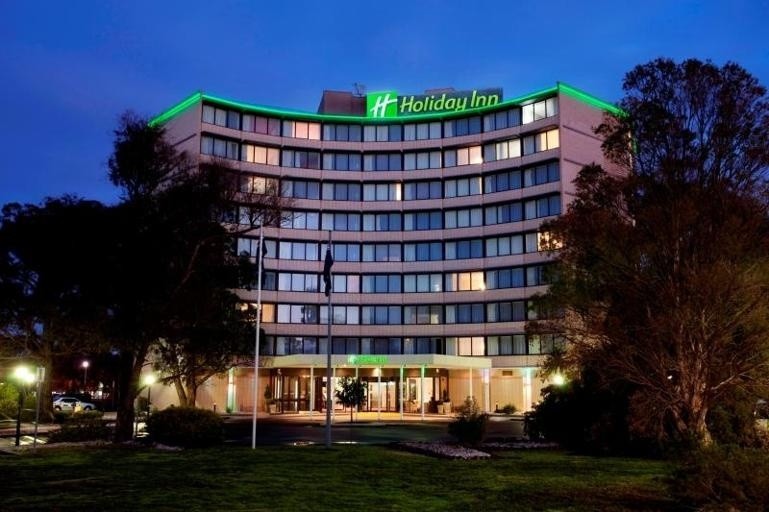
[53,395,97,412]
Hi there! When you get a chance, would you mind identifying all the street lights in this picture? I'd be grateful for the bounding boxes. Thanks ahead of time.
[81,359,90,393]
[144,374,156,419]
[10,365,35,447]
